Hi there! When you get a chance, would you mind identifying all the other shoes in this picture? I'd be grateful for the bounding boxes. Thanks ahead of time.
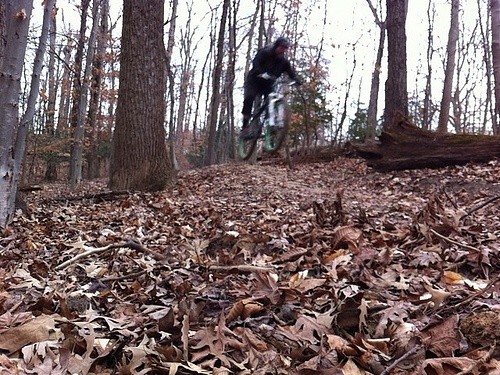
[240,126,252,137]
[268,126,277,135]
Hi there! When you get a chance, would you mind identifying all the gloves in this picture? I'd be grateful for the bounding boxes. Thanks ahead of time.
[259,72,270,81]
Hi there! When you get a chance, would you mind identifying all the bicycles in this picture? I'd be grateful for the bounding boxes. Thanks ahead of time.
[235,75,306,157]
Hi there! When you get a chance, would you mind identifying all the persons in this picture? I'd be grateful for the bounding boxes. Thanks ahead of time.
[241,37,306,133]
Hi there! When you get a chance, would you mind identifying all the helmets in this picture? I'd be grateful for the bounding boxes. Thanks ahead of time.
[274,38,289,49]
